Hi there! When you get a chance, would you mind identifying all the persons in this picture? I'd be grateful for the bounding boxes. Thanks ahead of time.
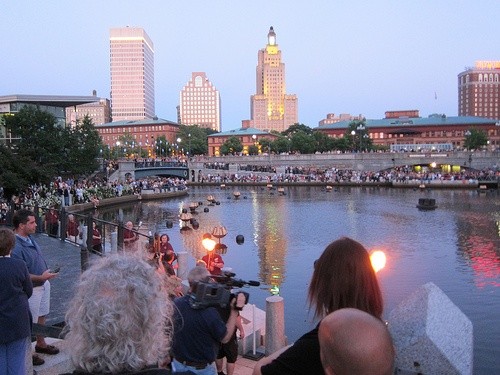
[214,308,245,375]
[0,228,33,375]
[319,306,397,375]
[166,266,246,375]
[0,146,500,275]
[56,245,198,375]
[253,238,383,375]
[9,209,59,366]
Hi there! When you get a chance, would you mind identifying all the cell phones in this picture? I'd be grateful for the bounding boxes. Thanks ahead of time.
[52,268,60,273]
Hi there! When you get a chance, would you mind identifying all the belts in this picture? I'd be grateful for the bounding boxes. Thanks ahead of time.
[174,355,214,369]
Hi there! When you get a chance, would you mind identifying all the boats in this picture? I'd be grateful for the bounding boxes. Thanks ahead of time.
[416,198,437,211]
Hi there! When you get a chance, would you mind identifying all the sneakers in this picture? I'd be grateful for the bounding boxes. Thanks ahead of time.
[32,354,46,366]
[35,343,60,355]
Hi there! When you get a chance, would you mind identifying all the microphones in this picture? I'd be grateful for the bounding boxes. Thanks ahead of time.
[245,280,260,286]
